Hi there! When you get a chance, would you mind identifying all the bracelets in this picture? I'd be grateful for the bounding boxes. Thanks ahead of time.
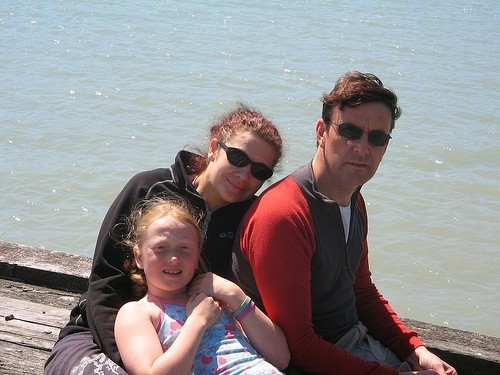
[235,300,256,322]
[231,296,251,317]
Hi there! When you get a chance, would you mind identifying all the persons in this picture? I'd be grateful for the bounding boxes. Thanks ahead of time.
[111,194,290,375]
[40,103,284,375]
[216,69,459,375]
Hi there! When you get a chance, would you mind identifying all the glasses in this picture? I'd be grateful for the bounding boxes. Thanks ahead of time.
[218,140,274,181]
[329,121,392,147]
